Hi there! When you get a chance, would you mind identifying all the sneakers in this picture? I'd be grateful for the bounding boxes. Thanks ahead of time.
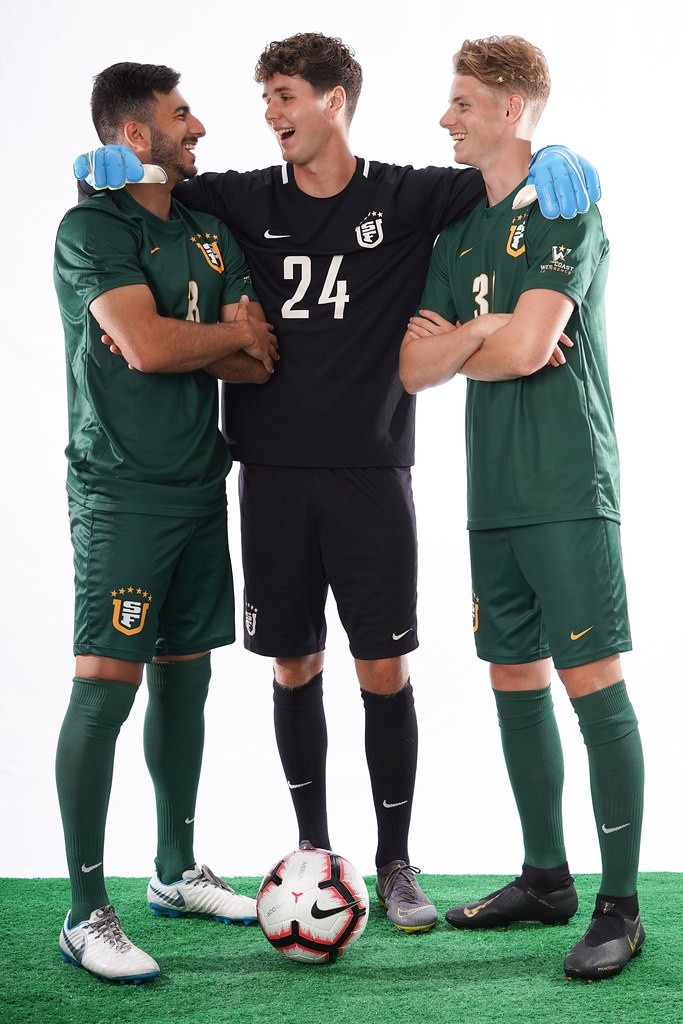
[147,866,259,926]
[375,861,439,935]
[564,898,645,982]
[59,905,160,985]
[445,874,578,929]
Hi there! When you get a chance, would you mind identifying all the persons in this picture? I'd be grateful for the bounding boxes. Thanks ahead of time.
[71,29,601,933]
[46,58,280,982]
[399,33,649,980]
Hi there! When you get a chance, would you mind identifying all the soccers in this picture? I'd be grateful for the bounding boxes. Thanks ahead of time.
[257,847,370,964]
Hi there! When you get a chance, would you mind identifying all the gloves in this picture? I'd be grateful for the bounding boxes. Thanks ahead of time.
[511,144,602,221]
[72,144,167,194]
